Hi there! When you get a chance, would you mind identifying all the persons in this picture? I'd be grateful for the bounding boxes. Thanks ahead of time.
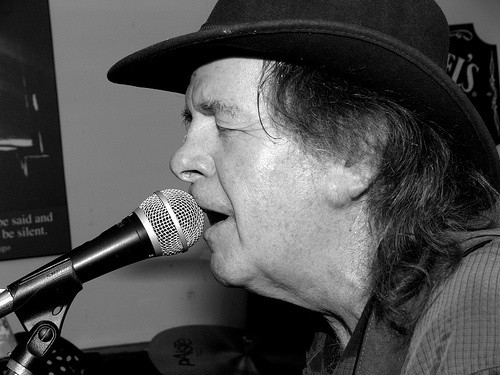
[108,0,500,375]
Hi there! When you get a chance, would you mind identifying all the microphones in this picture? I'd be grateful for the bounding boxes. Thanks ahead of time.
[0,189,204,318]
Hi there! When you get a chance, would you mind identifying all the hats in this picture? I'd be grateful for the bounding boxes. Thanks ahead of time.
[107,0,500,192]
